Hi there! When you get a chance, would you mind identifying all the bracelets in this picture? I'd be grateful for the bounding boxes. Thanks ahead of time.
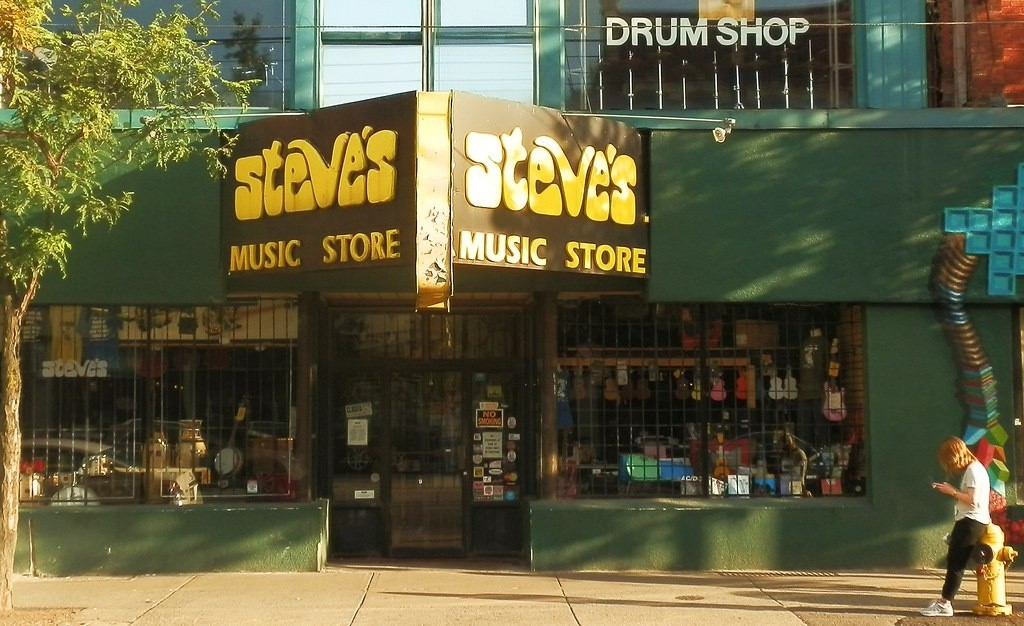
[952,490,957,497]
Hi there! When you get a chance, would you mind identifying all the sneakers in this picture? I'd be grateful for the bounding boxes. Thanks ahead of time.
[920,601,953,616]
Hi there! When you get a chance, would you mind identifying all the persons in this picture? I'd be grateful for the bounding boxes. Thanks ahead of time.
[920,436,990,616]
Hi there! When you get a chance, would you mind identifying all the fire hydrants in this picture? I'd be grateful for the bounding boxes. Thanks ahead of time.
[971,519,1018,615]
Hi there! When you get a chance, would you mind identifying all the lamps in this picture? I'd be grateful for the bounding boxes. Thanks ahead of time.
[140,112,305,137]
[562,111,736,144]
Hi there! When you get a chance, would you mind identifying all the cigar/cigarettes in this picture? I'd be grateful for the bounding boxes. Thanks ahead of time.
[933,485,937,488]
